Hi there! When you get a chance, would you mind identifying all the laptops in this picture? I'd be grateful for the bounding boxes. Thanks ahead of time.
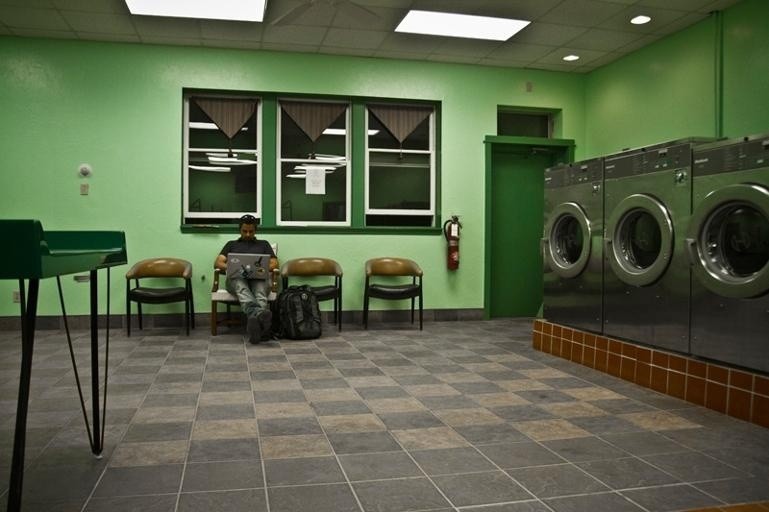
[227,253,270,280]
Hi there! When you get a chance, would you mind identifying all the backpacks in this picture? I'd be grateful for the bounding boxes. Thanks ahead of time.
[273,285,322,339]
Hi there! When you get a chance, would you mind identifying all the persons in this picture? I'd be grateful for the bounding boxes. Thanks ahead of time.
[214,215,279,343]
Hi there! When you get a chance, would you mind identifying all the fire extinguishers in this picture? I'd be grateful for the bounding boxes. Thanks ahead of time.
[444,215,463,270]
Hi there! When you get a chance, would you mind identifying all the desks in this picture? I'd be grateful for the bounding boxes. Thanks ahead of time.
[0,218,128,512]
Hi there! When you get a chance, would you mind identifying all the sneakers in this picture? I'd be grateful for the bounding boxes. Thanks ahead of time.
[261,310,273,334]
[246,317,262,344]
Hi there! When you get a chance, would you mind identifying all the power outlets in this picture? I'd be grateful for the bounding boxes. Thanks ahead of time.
[13,290,20,302]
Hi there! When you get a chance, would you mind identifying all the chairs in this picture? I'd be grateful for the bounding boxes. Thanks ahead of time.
[281,257,343,333]
[210,243,279,336]
[125,257,195,337]
[362,257,424,331]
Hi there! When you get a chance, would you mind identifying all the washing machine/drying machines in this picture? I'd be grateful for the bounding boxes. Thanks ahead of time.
[689,131,769,375]
[604,137,731,355]
[539,156,613,335]
